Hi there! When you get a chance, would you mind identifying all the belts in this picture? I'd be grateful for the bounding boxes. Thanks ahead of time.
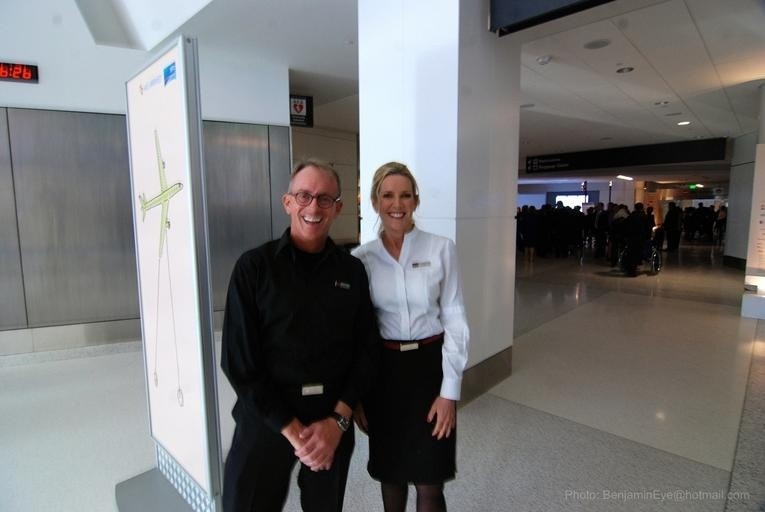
[293,384,335,398]
[384,336,444,353]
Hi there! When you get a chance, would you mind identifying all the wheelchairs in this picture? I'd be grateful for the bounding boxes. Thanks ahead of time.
[618,244,662,275]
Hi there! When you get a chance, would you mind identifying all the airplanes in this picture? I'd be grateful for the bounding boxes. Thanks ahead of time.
[138,128,184,259]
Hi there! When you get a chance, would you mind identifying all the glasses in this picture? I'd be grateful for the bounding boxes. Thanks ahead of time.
[288,191,343,210]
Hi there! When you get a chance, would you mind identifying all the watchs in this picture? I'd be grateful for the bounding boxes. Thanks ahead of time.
[330,412,350,431]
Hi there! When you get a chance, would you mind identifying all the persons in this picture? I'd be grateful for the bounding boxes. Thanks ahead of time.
[217,159,380,511]
[348,160,470,511]
[516,197,728,277]
